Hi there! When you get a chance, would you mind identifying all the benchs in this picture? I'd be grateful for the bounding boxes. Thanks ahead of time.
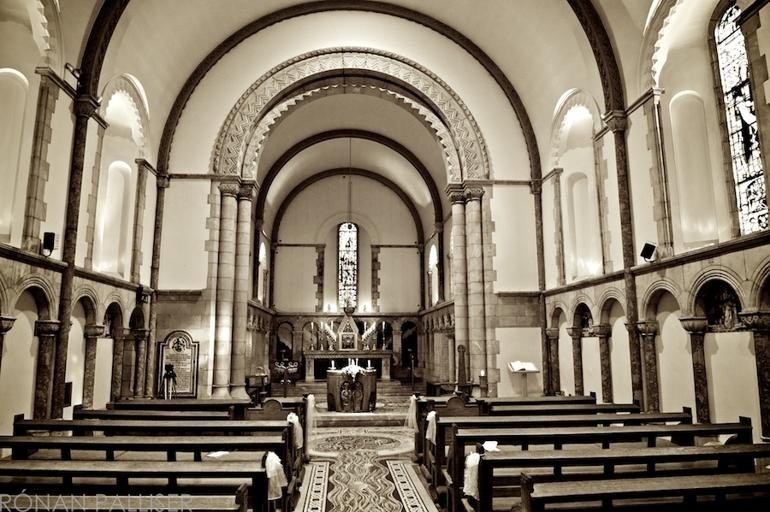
[414,391,770,511]
[1,394,307,512]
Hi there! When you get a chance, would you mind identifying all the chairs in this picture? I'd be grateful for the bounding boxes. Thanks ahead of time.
[244,374,272,410]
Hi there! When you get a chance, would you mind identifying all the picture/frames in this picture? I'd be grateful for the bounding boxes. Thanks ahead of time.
[156,330,200,399]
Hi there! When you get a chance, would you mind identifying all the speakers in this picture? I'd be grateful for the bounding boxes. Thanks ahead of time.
[639,242,657,259]
[42,232,55,250]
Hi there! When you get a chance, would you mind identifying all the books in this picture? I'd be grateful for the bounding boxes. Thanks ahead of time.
[510,360,537,372]
